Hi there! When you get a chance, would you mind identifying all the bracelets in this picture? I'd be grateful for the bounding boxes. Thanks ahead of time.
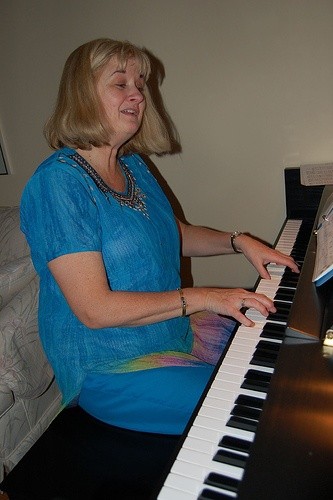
[176,287,186,318]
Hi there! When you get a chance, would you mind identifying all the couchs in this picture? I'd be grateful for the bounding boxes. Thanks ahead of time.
[0,204,65,494]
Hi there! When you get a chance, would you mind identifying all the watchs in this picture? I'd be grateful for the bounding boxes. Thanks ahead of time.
[231,231,243,254]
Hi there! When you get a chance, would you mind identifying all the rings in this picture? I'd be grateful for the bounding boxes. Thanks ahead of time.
[241,298,246,307]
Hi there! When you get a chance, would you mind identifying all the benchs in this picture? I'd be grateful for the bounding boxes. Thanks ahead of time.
[0,402,185,500]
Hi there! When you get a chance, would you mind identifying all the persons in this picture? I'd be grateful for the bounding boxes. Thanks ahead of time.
[19,38,301,435]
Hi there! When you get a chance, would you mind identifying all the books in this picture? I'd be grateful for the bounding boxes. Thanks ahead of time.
[312,189,333,287]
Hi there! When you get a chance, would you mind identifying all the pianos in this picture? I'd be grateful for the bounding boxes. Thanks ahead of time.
[156,161,333,500]
